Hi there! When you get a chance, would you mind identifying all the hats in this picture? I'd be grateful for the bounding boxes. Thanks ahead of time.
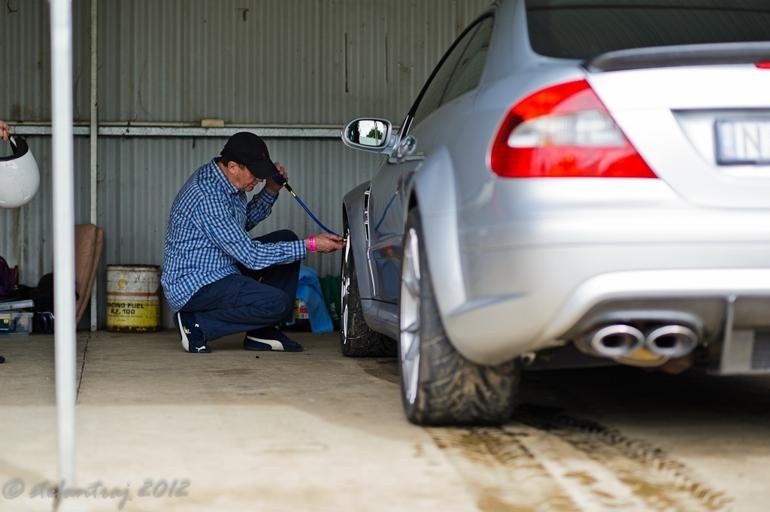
[225,132,277,179]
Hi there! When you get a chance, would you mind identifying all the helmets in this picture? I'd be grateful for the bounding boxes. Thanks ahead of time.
[0,135,40,208]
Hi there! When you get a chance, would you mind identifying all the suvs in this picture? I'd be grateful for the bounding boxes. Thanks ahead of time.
[341,0,769,426]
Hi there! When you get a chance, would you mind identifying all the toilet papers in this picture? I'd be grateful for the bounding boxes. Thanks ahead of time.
[200,118,225,128]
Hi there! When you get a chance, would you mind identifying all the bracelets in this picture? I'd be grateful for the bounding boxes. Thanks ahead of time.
[308,236,316,252]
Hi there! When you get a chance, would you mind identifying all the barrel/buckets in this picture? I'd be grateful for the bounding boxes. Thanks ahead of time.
[105,263,161,332]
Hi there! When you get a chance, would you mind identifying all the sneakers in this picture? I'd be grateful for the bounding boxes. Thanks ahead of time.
[173,311,212,353]
[243,330,304,352]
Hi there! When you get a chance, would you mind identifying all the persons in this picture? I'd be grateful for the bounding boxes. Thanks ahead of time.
[162,131,345,353]
[0,120,9,141]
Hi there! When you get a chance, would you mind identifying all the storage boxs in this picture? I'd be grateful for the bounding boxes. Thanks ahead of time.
[0,300,35,336]
